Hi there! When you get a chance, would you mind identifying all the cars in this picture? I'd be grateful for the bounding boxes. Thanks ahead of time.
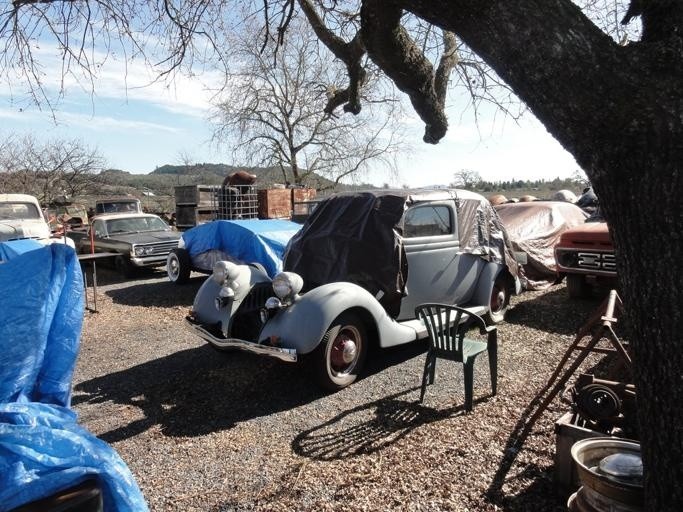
[552,197,620,300]
[181,185,518,393]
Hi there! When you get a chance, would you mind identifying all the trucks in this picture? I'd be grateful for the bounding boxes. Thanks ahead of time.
[0,193,74,264]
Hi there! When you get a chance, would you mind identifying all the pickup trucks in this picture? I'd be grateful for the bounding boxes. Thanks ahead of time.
[65,209,187,279]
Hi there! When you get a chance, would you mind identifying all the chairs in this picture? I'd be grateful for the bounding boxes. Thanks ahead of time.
[415,303,497,412]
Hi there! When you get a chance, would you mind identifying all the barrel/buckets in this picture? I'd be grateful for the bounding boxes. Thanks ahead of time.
[571,435,646,512]
